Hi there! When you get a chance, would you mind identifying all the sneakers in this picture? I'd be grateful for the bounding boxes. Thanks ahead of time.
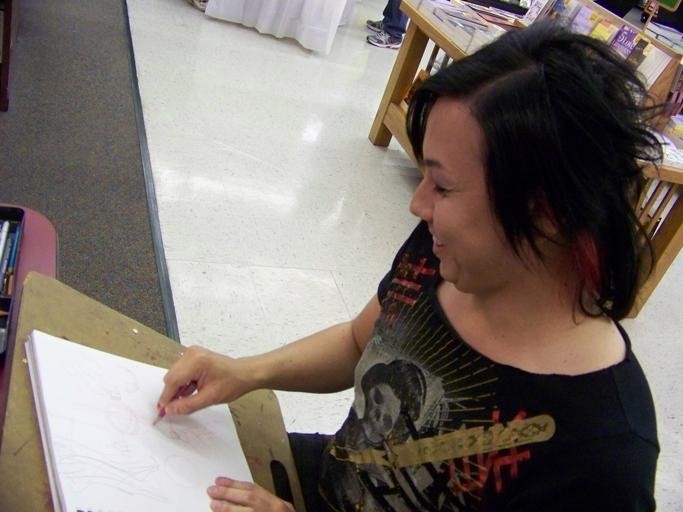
[367,32,402,49]
[367,20,382,33]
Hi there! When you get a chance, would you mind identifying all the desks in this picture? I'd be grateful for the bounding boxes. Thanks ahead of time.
[369,2,683,315]
[2,0,182,342]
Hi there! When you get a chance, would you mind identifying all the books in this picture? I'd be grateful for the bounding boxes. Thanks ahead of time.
[23,328,255,512]
[397,0,681,126]
[642,127,681,169]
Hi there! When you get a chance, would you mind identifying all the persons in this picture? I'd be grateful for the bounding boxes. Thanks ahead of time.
[154,24,658,512]
[363,0,410,48]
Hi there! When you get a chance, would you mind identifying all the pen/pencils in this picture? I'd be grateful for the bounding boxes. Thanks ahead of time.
[151,380,194,425]
[0,222,21,296]
